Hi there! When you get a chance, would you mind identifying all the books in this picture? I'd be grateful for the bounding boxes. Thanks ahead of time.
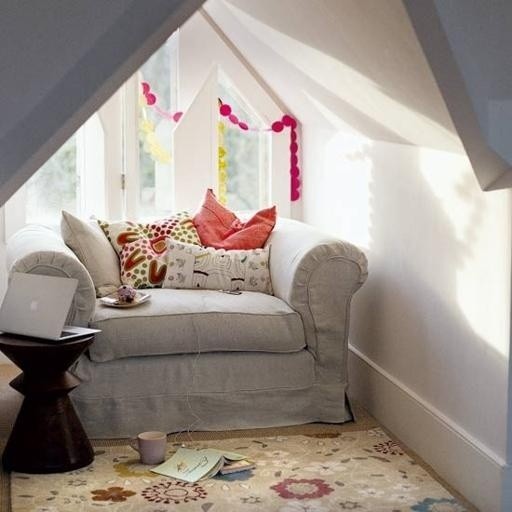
[150,447,251,483]
[220,458,257,475]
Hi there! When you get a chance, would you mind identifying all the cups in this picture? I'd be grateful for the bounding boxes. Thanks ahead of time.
[128,431,168,464]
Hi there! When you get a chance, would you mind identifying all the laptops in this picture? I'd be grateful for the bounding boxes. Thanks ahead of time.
[0,272,102,341]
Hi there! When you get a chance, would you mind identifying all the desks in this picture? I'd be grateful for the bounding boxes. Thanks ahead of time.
[0,333,94,474]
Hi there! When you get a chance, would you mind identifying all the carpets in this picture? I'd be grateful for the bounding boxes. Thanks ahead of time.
[11,427,470,512]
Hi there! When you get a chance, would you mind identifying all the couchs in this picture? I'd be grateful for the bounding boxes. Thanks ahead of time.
[4,216,369,441]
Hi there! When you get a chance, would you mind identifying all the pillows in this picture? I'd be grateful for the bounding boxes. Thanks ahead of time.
[96,188,276,295]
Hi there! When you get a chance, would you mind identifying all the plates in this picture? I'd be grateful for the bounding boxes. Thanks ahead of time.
[100,291,152,309]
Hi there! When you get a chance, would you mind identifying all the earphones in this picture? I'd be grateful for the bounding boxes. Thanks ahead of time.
[181,444,185,448]
